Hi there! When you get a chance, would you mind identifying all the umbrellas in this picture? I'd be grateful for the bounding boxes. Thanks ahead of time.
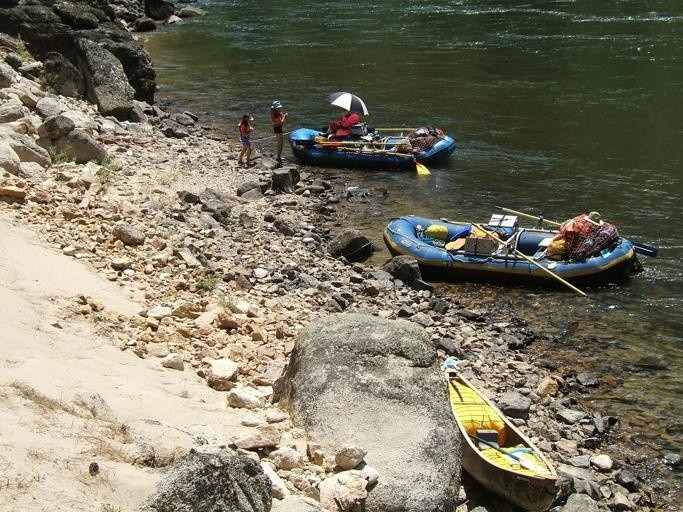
[326,90,370,116]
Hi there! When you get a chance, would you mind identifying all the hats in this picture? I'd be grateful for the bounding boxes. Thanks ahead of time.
[584,212,603,226]
[271,100,282,108]
[245,114,254,120]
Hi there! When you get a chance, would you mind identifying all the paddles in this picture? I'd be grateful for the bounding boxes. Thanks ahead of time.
[415,160,431,174]
[469,432,550,475]
[494,205,658,259]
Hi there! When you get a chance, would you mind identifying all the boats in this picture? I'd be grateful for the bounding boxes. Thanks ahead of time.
[383,215,635,290]
[291,128,455,168]
[446,367,558,512]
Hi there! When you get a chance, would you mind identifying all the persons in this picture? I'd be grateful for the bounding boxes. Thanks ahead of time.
[270,100,288,164]
[237,113,254,165]
[318,111,362,141]
